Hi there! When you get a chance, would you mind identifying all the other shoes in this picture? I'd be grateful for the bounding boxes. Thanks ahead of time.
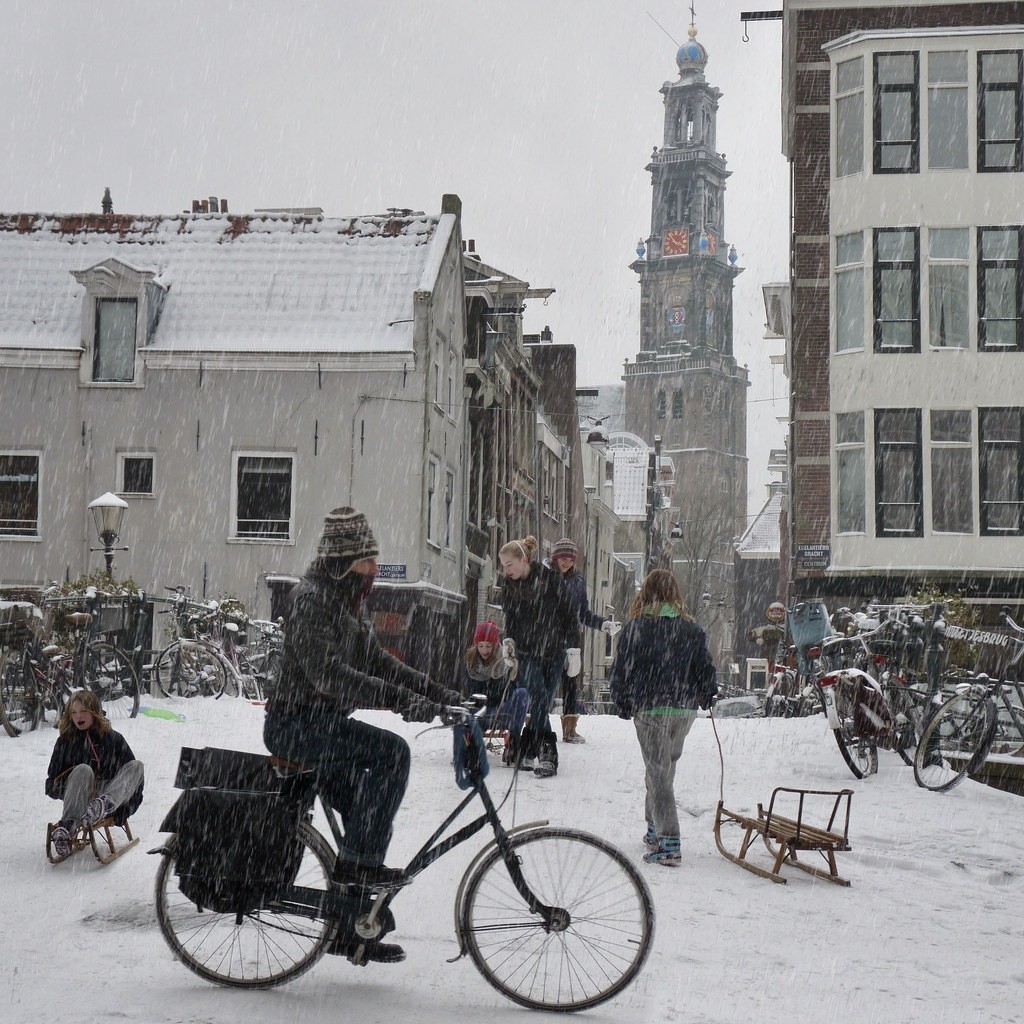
[643,834,660,850]
[331,857,406,884]
[643,848,681,865]
[326,935,406,962]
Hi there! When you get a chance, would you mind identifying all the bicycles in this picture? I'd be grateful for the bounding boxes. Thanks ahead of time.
[0,605,140,737]
[156,693,653,1013]
[914,607,1024,792]
[153,586,283,699]
[762,613,921,778]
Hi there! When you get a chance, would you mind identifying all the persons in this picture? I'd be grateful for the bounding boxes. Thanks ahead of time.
[451,619,529,764]
[498,536,583,776]
[526,538,623,743]
[46,690,144,856]
[606,569,718,869]
[263,506,473,963]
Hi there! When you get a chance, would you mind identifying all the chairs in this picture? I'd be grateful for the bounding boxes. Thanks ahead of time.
[46,815,139,864]
[716,787,854,887]
[789,603,844,674]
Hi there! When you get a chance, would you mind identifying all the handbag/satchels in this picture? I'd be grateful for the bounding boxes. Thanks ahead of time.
[175,787,295,914]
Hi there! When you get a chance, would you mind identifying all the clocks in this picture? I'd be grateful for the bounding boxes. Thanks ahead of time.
[663,229,688,256]
[708,233,717,254]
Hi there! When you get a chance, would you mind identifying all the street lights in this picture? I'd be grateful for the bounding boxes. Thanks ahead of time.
[87,492,129,662]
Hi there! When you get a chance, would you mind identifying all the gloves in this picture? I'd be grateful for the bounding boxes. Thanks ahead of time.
[564,647,581,677]
[395,688,437,723]
[617,702,632,721]
[602,620,623,635]
[502,638,516,668]
[700,695,712,710]
[427,681,465,707]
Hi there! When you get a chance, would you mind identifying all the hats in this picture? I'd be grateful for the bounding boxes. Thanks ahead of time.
[475,620,500,646]
[551,538,578,560]
[318,507,379,581]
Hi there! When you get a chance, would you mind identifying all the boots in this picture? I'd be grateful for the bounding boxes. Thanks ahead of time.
[519,727,537,770]
[80,795,114,828]
[533,733,558,776]
[560,713,586,744]
[51,819,77,857]
